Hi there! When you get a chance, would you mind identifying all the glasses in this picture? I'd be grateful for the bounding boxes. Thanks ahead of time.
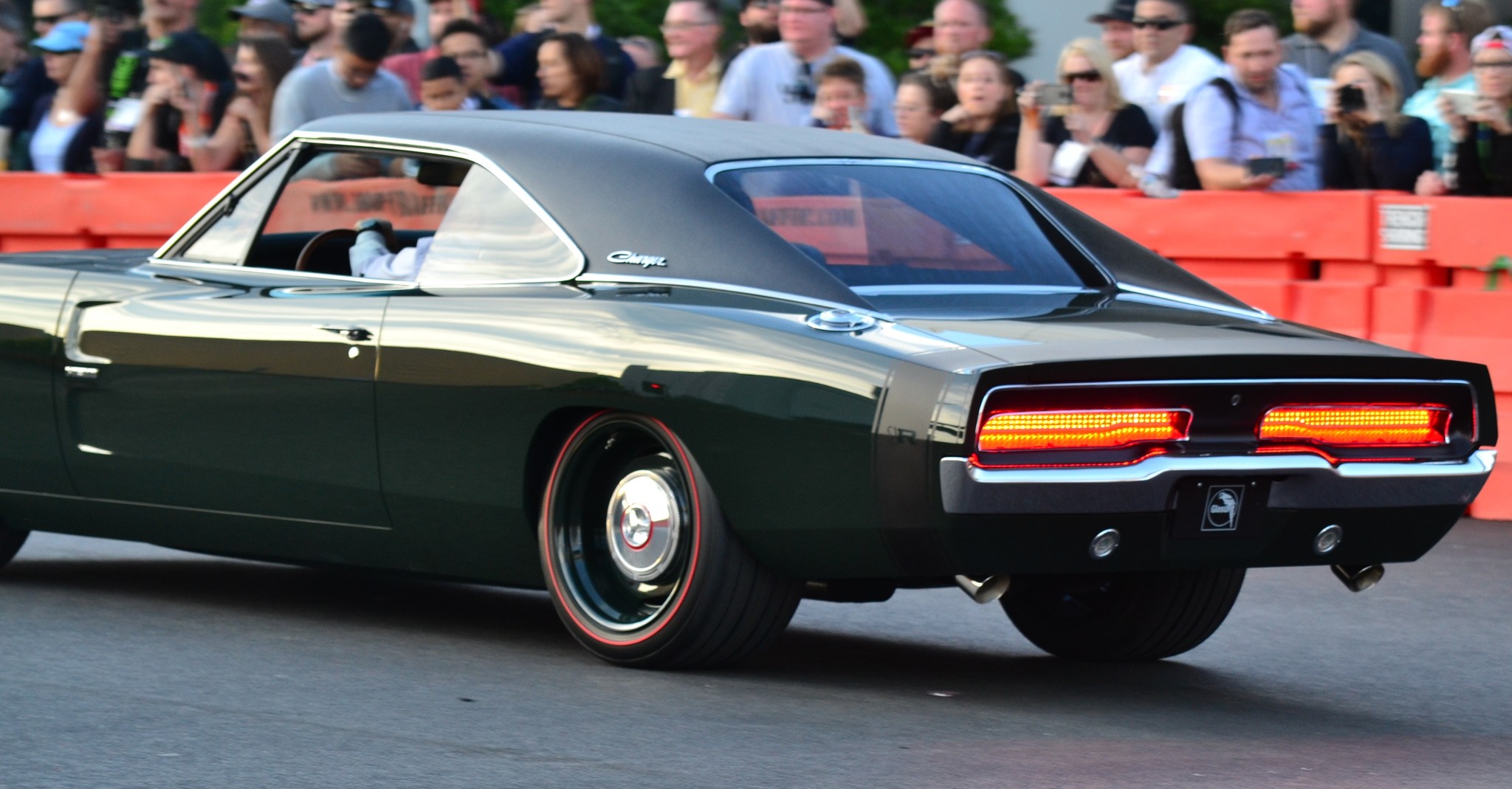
[1060,68,1101,88]
[908,47,941,60]
[958,49,1004,66]
[27,13,75,27]
[659,19,720,34]
[449,50,486,61]
[297,6,323,17]
[1131,16,1185,31]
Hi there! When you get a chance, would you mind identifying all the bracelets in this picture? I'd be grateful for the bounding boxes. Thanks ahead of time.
[1085,135,1101,148]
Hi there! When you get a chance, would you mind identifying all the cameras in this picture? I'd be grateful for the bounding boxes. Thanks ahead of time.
[1338,88,1366,111]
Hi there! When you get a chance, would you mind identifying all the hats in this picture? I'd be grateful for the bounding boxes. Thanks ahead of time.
[226,1,292,25]
[364,0,414,16]
[143,30,221,67]
[1088,1,1137,24]
[1470,25,1511,56]
[29,21,91,51]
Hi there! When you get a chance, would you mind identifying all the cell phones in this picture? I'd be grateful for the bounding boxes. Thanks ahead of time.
[1435,90,1483,116]
[1250,157,1286,179]
[1036,85,1073,103]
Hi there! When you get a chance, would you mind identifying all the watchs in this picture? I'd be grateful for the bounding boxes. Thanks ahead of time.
[358,219,385,235]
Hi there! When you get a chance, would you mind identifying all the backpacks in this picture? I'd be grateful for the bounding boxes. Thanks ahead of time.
[1167,79,1240,189]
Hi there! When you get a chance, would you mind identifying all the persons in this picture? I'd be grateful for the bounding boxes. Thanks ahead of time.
[0,0,1512,196]
[349,217,434,286]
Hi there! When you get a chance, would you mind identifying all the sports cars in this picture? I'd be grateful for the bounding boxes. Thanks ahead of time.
[0,107,1502,675]
